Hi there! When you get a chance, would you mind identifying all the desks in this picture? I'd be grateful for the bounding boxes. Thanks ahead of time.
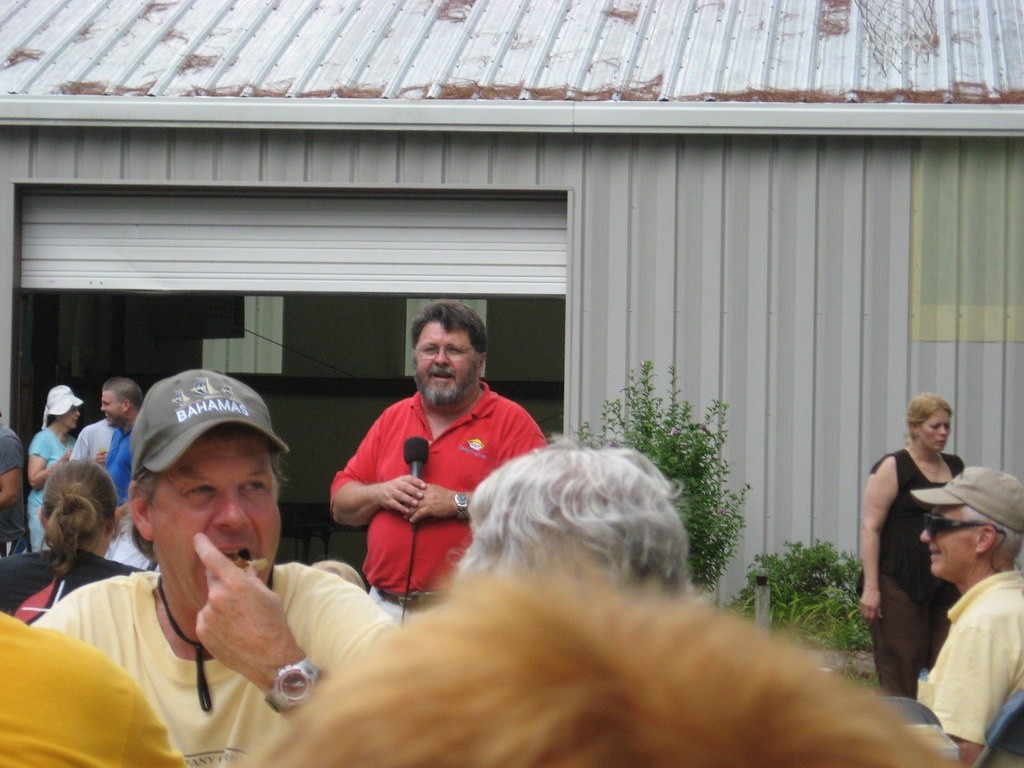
[912,722,962,760]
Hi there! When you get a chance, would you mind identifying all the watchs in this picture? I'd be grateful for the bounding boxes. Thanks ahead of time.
[264,658,319,713]
[453,492,469,519]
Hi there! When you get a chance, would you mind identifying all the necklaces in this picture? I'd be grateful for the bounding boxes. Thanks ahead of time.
[158,565,274,712]
[923,461,941,483]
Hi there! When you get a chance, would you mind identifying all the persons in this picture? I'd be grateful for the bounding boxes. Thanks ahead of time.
[910,465,1024,768]
[858,395,965,701]
[454,441,691,607]
[31,370,396,768]
[0,460,146,626]
[328,303,547,625]
[0,376,149,570]
[311,560,366,590]
[0,610,186,768]
[259,585,954,768]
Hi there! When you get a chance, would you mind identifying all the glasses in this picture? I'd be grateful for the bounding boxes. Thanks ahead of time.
[415,343,474,359]
[925,513,1006,537]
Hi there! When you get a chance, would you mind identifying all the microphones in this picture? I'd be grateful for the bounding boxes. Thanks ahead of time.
[403,437,429,531]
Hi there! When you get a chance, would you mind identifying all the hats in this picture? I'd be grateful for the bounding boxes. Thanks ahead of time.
[130,369,290,480]
[910,467,1024,533]
[41,385,84,429]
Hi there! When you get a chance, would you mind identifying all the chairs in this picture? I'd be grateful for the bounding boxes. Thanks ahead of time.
[969,690,1024,768]
[878,696,944,730]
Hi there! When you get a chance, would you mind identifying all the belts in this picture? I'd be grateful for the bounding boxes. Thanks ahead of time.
[377,588,444,608]
